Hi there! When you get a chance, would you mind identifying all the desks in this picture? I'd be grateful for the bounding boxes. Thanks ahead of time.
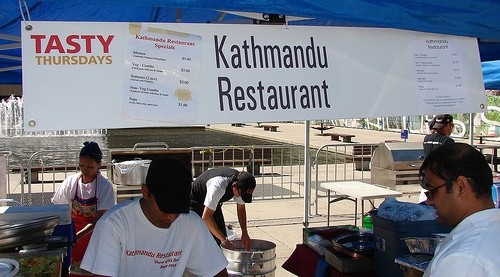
[320,181,402,227]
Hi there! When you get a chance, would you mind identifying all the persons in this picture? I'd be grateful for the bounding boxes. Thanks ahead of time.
[51,142,115,277]
[79,155,230,277]
[191,166,256,251]
[423,114,455,161]
[418,143,500,277]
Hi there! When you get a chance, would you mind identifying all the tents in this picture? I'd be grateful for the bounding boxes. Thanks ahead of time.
[469,60,500,147]
[0,0,500,229]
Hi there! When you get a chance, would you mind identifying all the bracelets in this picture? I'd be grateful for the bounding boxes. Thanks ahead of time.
[221,237,228,243]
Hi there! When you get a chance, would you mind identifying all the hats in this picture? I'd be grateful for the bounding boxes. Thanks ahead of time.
[432,114,453,129]
[237,171,256,203]
[145,157,193,214]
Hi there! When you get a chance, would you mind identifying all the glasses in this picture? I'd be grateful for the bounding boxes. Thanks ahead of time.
[451,124,454,127]
[424,177,472,200]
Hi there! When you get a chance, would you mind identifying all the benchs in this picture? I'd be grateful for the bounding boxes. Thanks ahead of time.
[190,145,274,177]
[261,125,279,131]
[12,164,109,184]
[231,123,246,127]
[346,143,379,172]
[326,133,356,142]
